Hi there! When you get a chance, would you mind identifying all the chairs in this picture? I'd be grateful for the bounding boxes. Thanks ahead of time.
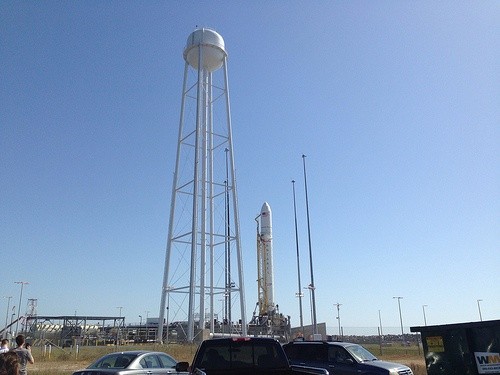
[257,353,275,375]
[145,360,152,368]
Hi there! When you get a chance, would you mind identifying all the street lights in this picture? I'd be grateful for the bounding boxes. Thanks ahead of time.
[392,295,405,336]
[476,299,483,322]
[13,281,30,344]
[422,304,429,327]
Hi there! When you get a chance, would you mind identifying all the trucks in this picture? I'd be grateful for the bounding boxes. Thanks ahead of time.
[409,319,500,375]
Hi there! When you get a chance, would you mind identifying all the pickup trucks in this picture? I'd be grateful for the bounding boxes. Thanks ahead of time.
[175,336,330,375]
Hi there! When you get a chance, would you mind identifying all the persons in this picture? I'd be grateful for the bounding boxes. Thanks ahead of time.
[0,335,34,375]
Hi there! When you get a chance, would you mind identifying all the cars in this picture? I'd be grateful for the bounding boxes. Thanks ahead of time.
[71,351,189,375]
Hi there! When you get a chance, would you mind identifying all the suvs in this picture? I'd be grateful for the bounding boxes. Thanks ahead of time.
[277,336,413,375]
